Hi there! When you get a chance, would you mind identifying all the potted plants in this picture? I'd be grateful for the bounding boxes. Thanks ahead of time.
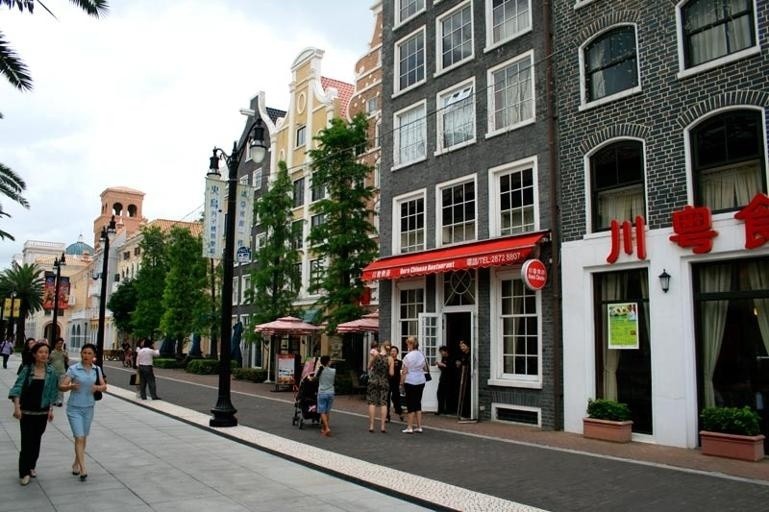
[583,398,634,443]
[698,406,766,463]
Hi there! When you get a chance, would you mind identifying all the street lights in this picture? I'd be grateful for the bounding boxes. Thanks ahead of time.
[9,289,17,337]
[207,119,266,426]
[96,216,116,385]
[48,252,67,349]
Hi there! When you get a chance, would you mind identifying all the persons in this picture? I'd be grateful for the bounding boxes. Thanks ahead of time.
[0,336,162,405]
[57,344,107,479]
[366,336,470,433]
[308,355,336,436]
[8,344,60,486]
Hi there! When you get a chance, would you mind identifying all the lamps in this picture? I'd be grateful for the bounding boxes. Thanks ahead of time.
[658,268,671,293]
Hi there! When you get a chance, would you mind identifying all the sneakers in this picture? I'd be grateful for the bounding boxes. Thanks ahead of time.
[21,469,36,485]
[319,428,330,435]
[72,464,88,480]
[402,429,422,434]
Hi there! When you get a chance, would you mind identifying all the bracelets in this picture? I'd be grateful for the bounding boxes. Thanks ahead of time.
[49,407,53,411]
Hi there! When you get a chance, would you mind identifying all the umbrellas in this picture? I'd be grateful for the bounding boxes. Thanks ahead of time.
[254,316,321,336]
[334,310,380,374]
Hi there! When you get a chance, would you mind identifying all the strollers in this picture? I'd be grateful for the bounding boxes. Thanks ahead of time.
[293,373,322,428]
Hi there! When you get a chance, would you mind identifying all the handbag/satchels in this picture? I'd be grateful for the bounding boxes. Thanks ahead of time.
[359,373,369,384]
[130,375,141,385]
[424,372,432,381]
[94,375,102,401]
